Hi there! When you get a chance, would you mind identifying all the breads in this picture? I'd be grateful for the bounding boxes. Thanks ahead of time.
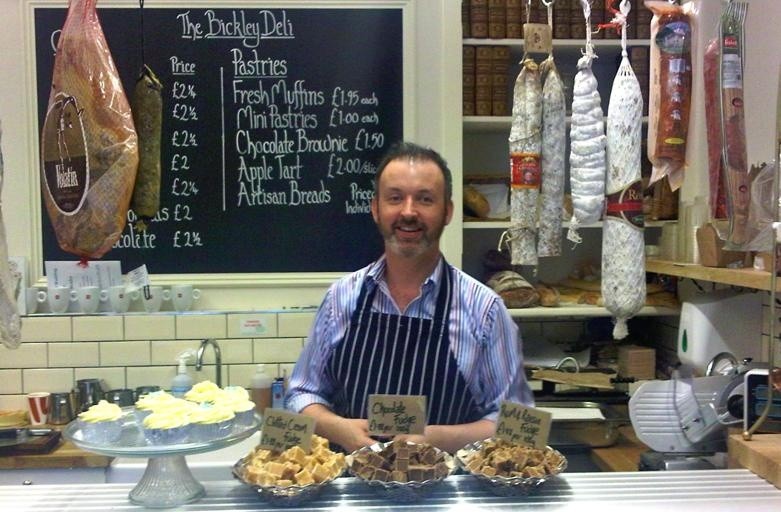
[477,266,676,309]
[462,183,490,219]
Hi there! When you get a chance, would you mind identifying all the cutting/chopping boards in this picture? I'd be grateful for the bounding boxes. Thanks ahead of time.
[0,408,114,468]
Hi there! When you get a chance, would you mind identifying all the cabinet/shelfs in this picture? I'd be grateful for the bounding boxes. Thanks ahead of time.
[440,1,683,409]
[586,258,781,472]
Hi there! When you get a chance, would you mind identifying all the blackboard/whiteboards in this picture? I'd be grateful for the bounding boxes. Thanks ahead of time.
[21,0,415,287]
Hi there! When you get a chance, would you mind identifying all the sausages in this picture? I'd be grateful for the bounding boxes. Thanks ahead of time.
[132,69,163,233]
[702,37,751,218]
[656,10,691,161]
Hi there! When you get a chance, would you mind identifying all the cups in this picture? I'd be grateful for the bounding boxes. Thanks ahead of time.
[26,378,161,425]
[37,284,201,312]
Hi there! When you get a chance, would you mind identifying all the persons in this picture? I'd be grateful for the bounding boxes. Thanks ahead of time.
[278,142,540,459]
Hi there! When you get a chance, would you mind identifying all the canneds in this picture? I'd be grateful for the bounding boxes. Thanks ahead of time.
[462,0,652,116]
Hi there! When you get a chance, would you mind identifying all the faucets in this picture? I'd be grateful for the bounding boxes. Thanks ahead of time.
[194,338,221,390]
[705,351,738,378]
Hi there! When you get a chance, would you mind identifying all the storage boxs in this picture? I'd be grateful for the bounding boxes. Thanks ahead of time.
[617,345,657,381]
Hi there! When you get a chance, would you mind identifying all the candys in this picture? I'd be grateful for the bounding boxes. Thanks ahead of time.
[352,441,450,481]
[243,432,345,486]
[463,440,563,479]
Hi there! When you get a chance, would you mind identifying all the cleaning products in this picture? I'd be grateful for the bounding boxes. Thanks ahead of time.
[247,363,273,415]
[169,349,195,399]
